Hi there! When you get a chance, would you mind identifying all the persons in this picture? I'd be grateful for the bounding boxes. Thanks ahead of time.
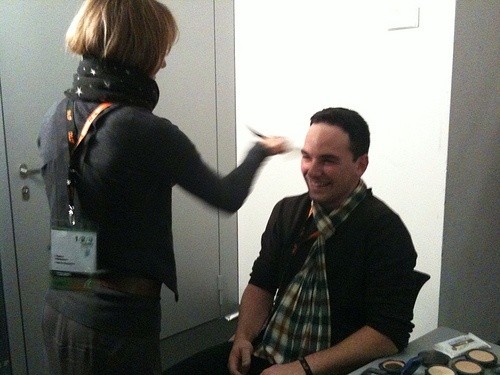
[161,108,418,375]
[37,0,300,375]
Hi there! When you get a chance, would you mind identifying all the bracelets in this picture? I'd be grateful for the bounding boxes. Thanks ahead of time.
[298,356,313,375]
